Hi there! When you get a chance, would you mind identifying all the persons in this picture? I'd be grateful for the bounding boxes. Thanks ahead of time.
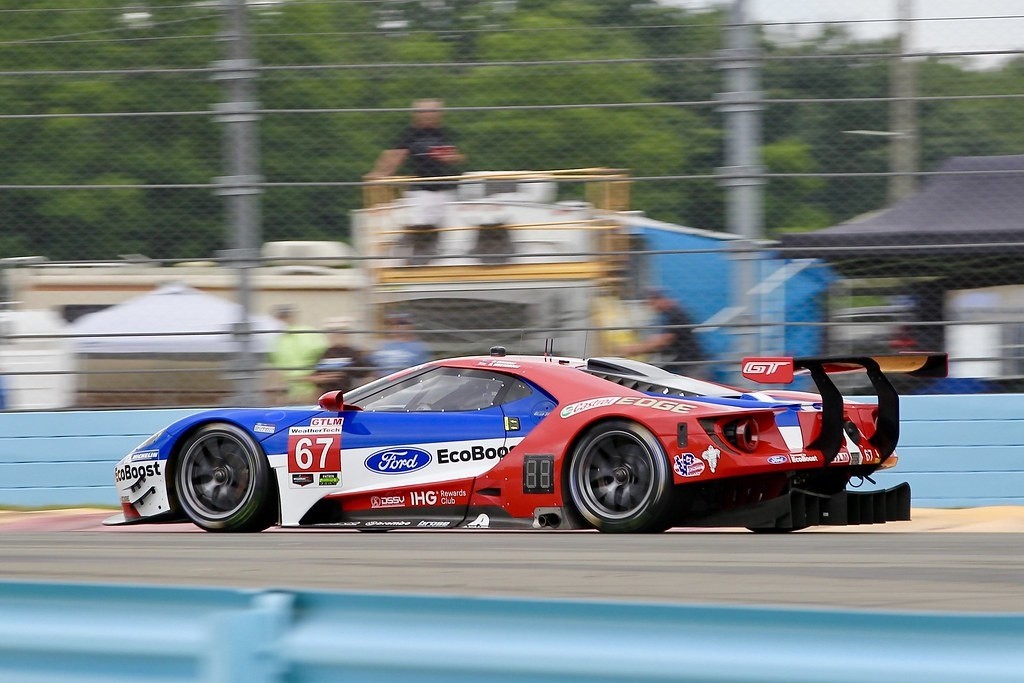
[264,298,435,406]
[607,280,713,381]
[363,91,470,204]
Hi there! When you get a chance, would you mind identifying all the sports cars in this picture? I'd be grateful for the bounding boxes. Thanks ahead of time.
[100,346,914,535]
